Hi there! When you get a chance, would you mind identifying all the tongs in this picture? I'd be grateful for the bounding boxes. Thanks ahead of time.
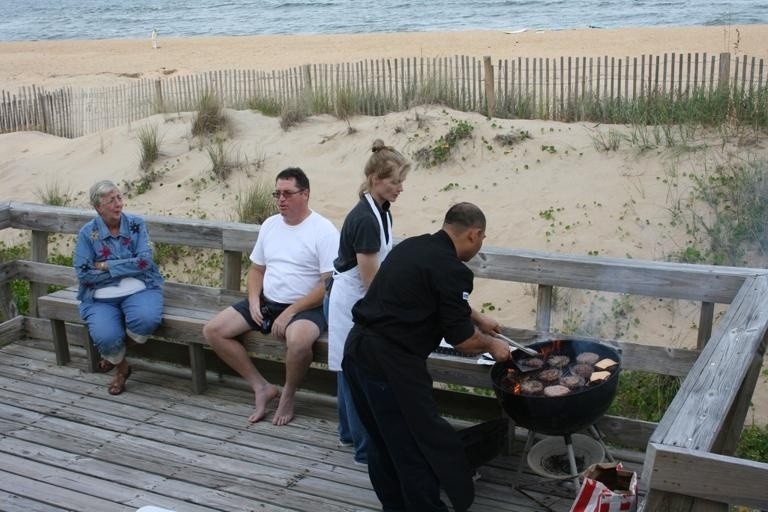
[489,330,542,357]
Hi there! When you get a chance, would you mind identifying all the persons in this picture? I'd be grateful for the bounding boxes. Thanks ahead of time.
[327,140,411,468]
[203,167,340,425]
[73,181,165,396]
[342,203,511,512]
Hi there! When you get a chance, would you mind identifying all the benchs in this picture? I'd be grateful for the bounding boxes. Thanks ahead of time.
[38,282,515,467]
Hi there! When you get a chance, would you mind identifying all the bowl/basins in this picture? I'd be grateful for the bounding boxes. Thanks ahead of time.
[526,433,604,482]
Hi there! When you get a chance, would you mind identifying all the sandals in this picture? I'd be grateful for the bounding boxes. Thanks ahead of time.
[97,360,114,372]
[109,366,131,395]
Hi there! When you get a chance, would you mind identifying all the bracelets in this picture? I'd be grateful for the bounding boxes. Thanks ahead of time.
[100,263,105,270]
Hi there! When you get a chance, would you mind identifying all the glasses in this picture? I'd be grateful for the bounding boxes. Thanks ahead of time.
[272,190,303,197]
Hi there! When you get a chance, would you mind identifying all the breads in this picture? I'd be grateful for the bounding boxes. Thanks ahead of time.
[519,352,617,397]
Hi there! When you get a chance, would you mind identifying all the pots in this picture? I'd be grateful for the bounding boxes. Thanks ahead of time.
[489,339,621,437]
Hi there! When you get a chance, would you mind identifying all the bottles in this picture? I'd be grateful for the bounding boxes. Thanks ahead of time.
[259,302,271,334]
